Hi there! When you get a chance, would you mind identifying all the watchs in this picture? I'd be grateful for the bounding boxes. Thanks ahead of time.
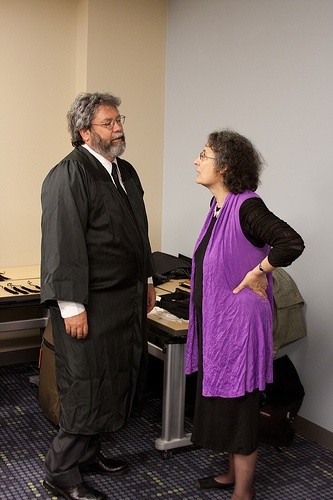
[259,262,268,275]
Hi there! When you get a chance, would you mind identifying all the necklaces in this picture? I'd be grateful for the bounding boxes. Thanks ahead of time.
[214,203,221,218]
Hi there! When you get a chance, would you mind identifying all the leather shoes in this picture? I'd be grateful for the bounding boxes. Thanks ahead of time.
[78,452,129,476]
[42,475,109,500]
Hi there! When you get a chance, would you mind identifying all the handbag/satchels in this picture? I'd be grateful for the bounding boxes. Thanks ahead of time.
[37,319,62,429]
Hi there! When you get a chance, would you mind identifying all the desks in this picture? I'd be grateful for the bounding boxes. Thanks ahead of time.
[0,252,190,457]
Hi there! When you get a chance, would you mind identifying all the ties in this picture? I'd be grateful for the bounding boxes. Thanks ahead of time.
[111,163,127,202]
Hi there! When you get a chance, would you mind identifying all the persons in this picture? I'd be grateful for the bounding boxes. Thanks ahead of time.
[182,131,305,500]
[43,93,156,500]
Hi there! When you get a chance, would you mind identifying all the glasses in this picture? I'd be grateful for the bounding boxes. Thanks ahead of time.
[90,115,126,130]
[200,151,221,162]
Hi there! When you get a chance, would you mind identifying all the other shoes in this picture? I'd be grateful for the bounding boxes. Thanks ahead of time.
[195,476,236,489]
